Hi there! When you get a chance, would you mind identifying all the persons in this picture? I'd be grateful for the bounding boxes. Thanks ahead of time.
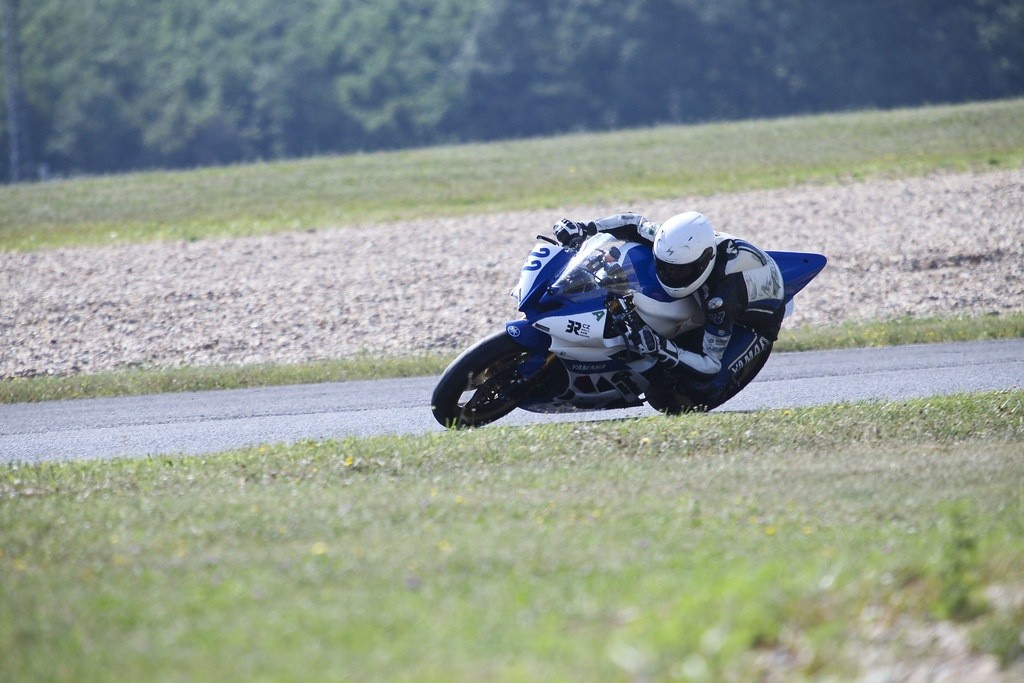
[554,210,784,414]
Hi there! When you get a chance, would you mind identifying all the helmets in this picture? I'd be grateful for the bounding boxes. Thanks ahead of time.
[652,211,716,299]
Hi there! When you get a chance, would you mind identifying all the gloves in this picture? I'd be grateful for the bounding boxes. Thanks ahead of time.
[552,218,598,248]
[628,322,661,357]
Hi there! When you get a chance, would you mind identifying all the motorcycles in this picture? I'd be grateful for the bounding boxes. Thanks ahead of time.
[430,231,829,431]
